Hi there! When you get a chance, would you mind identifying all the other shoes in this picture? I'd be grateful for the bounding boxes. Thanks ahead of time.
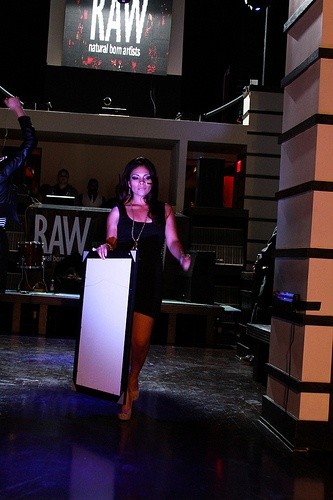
[119,386,139,421]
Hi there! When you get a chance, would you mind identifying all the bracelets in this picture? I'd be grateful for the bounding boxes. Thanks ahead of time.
[105,243,114,250]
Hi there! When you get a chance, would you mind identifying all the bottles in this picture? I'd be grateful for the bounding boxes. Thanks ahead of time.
[49,280,55,292]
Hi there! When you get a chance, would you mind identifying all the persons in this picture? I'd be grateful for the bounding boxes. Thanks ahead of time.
[0,97,38,297]
[41,169,77,206]
[96,156,192,420]
[14,183,35,215]
[78,178,103,207]
[247,226,276,381]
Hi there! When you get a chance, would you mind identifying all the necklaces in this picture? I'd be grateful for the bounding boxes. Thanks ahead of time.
[128,200,148,263]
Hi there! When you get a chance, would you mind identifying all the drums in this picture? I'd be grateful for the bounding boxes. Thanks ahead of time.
[16,241,45,269]
